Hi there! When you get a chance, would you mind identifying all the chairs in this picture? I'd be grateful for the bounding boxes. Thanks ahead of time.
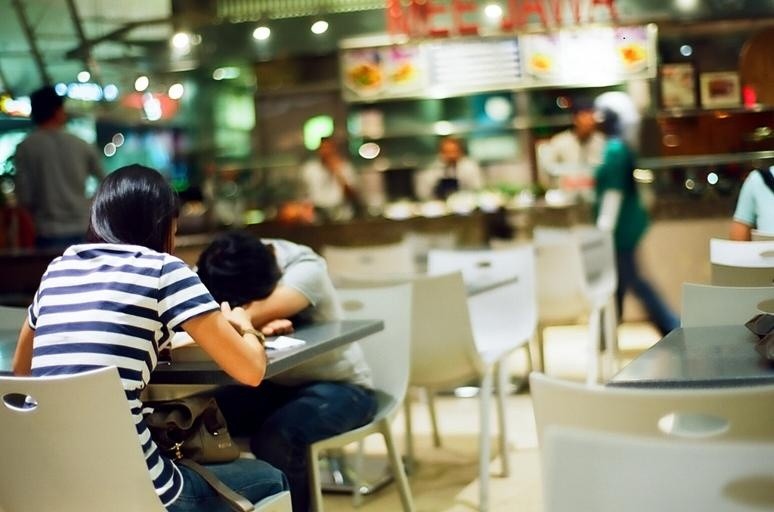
[0,221,774,512]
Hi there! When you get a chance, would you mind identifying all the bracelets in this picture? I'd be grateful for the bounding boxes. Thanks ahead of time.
[237,326,268,344]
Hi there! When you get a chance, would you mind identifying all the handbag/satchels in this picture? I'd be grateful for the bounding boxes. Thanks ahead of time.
[142,383,241,465]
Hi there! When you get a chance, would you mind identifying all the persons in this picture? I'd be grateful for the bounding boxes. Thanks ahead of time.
[15,160,284,512]
[724,158,773,247]
[288,136,376,220]
[192,229,379,512]
[409,132,490,201]
[12,83,107,240]
[534,105,600,206]
[585,91,679,345]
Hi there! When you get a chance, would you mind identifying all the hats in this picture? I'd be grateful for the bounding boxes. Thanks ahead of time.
[31,87,66,123]
